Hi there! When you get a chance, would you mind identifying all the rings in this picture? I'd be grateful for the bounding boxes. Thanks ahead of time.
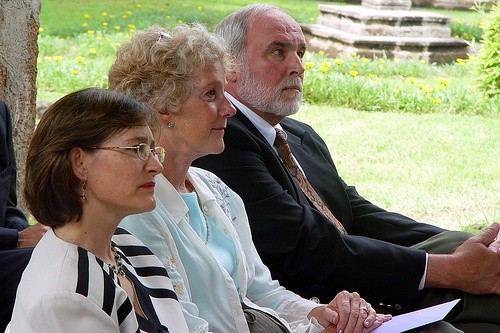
[359,307,369,316]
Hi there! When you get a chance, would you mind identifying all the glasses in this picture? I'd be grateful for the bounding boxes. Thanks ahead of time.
[66,143,166,164]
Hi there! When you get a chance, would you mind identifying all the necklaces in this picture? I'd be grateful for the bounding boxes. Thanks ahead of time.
[95,243,125,277]
[187,178,210,245]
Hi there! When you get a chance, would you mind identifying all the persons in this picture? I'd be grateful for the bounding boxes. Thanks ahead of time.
[109,23,465,333]
[190,3,500,325]
[0,98,51,333]
[4,87,190,333]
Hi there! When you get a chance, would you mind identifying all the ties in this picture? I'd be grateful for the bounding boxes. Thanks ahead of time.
[273,128,348,236]
